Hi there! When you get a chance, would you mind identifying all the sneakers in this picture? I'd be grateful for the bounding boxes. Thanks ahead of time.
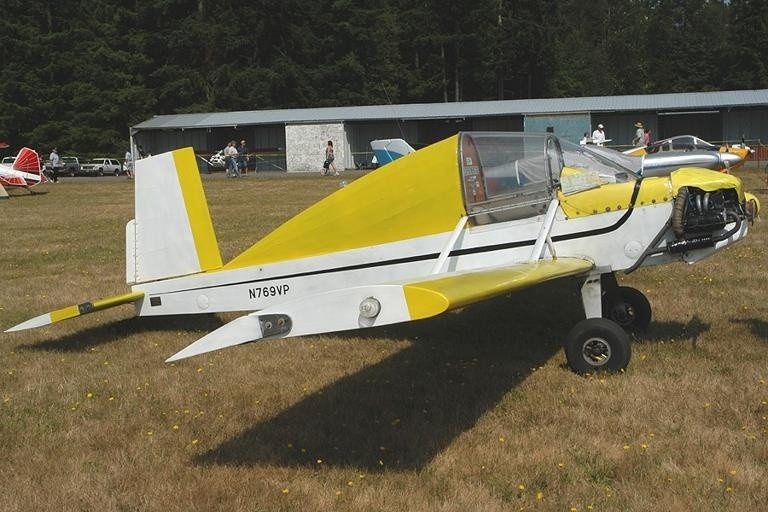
[333,172,339,177]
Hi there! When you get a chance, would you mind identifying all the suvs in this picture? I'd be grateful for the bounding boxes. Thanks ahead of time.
[2,156,122,176]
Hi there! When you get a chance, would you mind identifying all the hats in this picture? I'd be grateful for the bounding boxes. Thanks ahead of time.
[598,124,604,129]
[634,122,643,127]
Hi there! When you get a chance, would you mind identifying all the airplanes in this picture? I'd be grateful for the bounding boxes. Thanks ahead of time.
[194,149,285,173]
[0,147,47,198]
[1,131,764,382]
[368,138,741,199]
[622,135,756,173]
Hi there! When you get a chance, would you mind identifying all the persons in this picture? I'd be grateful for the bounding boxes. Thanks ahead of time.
[122,146,154,180]
[592,124,606,147]
[632,122,646,146]
[580,131,590,147]
[223,141,249,178]
[323,141,340,177]
[643,130,652,144]
[48,146,61,183]
